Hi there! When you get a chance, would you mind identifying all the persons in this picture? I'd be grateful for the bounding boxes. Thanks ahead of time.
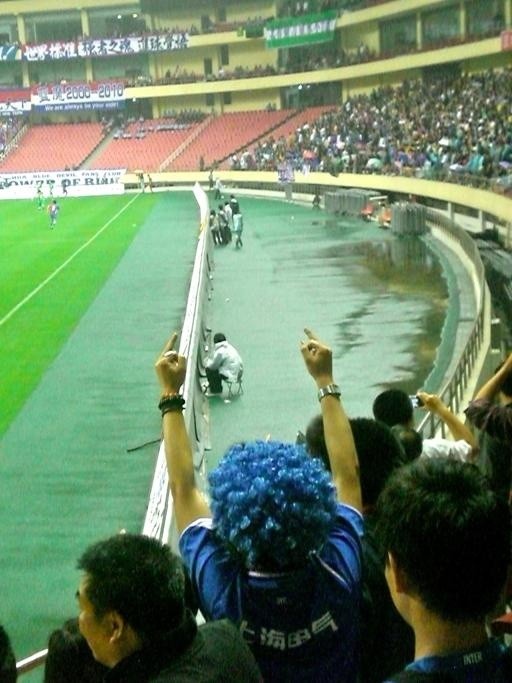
[372,387,481,464]
[223,201,233,221]
[208,210,222,245]
[154,324,369,680]
[217,204,231,243]
[317,383,341,401]
[378,204,392,226]
[199,332,245,396]
[231,209,244,248]
[464,350,512,506]
[156,392,186,415]
[46,199,60,229]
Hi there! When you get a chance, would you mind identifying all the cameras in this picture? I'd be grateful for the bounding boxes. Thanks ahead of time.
[408,395,424,408]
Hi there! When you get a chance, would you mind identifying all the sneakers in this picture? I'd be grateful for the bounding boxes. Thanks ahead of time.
[214,240,243,247]
[203,384,221,396]
